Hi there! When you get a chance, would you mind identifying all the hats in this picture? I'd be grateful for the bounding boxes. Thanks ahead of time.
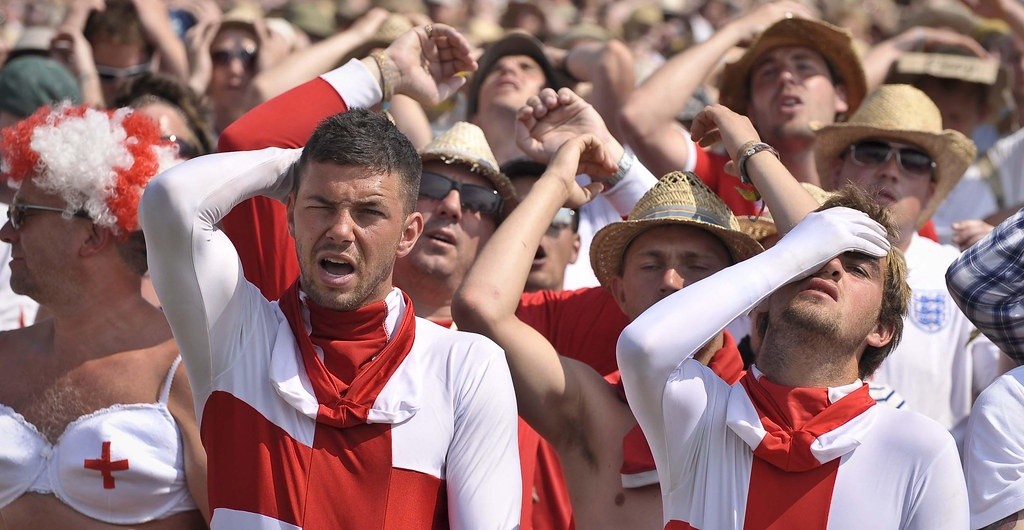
[468,29,560,115]
[9,25,59,51]
[337,13,413,67]
[417,121,515,201]
[807,83,978,231]
[623,6,662,29]
[0,57,83,118]
[221,2,262,32]
[589,170,765,289]
[895,43,1001,85]
[719,15,868,123]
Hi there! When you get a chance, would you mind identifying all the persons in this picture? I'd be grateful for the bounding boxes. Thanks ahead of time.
[140,108,522,530]
[0,2,1024,530]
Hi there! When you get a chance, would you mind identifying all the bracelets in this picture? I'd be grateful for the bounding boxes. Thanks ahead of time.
[737,140,781,187]
[371,48,393,104]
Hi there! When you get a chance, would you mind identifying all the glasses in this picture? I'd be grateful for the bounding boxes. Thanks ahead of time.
[94,64,146,83]
[6,203,92,231]
[551,207,577,226]
[211,37,257,66]
[850,137,938,175]
[418,171,502,215]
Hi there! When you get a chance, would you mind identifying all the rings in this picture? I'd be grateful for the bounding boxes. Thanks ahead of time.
[426,24,434,34]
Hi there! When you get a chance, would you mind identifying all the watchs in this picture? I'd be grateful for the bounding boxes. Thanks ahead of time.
[592,140,635,192]
